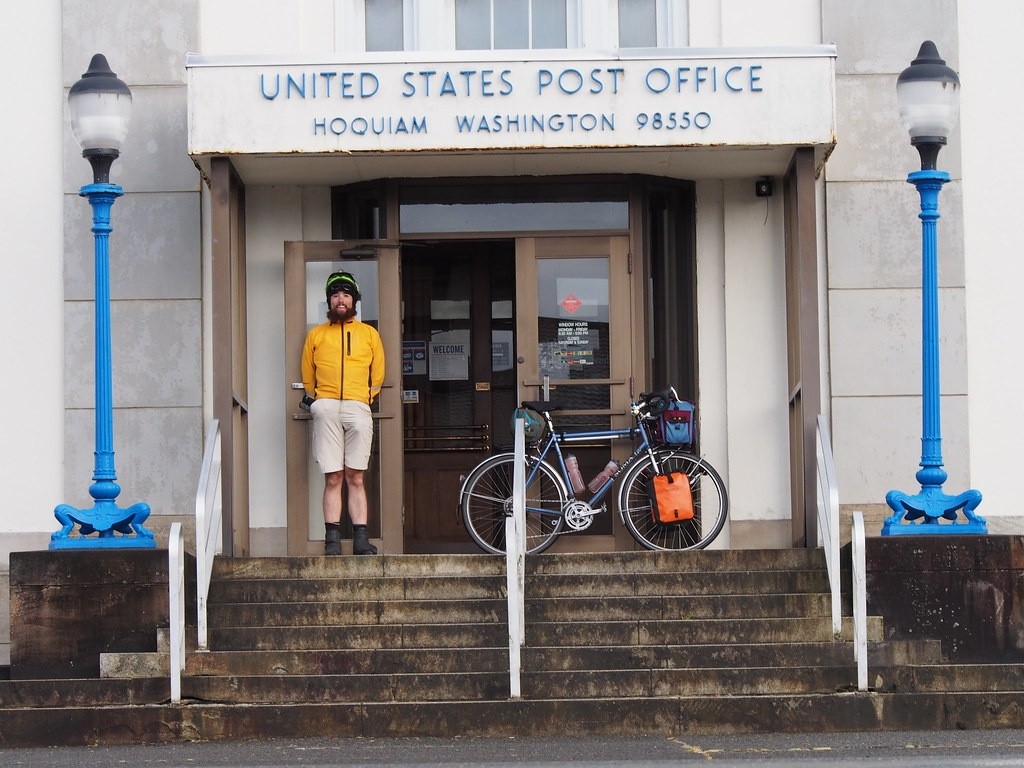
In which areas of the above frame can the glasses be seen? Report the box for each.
[331,284,352,293]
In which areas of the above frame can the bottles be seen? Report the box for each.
[564,452,586,494]
[588,459,621,494]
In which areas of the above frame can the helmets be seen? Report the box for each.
[325,271,361,301]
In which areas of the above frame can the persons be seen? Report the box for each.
[300,271,386,555]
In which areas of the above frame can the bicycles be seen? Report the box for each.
[457,385,728,556]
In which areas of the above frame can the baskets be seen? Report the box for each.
[651,398,699,445]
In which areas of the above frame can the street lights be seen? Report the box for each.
[52,53,157,549]
[881,40,987,536]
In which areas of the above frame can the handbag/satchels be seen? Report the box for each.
[646,468,695,524]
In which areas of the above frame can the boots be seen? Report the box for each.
[353,523,377,555]
[325,522,342,555]
